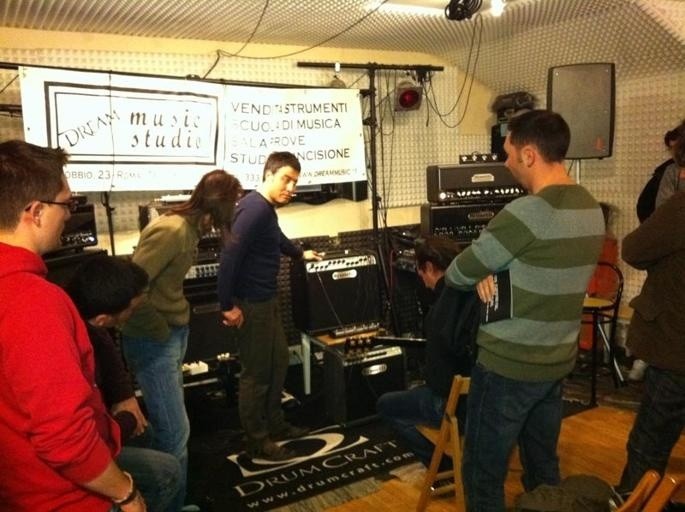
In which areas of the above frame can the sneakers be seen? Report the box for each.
[269,424,309,441]
[245,443,293,461]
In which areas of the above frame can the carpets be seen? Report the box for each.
[562,371,683,435]
[178,383,597,512]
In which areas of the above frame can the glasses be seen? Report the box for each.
[25,197,77,215]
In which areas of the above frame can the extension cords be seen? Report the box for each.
[328,320,380,339]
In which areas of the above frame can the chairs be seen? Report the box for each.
[414,375,471,512]
[567,262,623,406]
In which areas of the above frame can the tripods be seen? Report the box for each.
[576,159,629,387]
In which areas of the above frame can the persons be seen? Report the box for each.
[0,139,147,512]
[446,109,608,512]
[115,168,242,510]
[58,253,197,511]
[607,138,685,506]
[636,129,677,221]
[217,151,323,462]
[375,236,488,497]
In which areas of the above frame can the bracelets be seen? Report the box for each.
[111,470,137,505]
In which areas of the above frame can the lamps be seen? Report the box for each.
[395,78,423,112]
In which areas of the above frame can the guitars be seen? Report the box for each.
[344,337,426,354]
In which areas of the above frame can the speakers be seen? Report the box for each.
[289,250,382,337]
[323,340,405,430]
[546,63,615,160]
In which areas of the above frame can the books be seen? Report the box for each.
[477,268,512,326]
